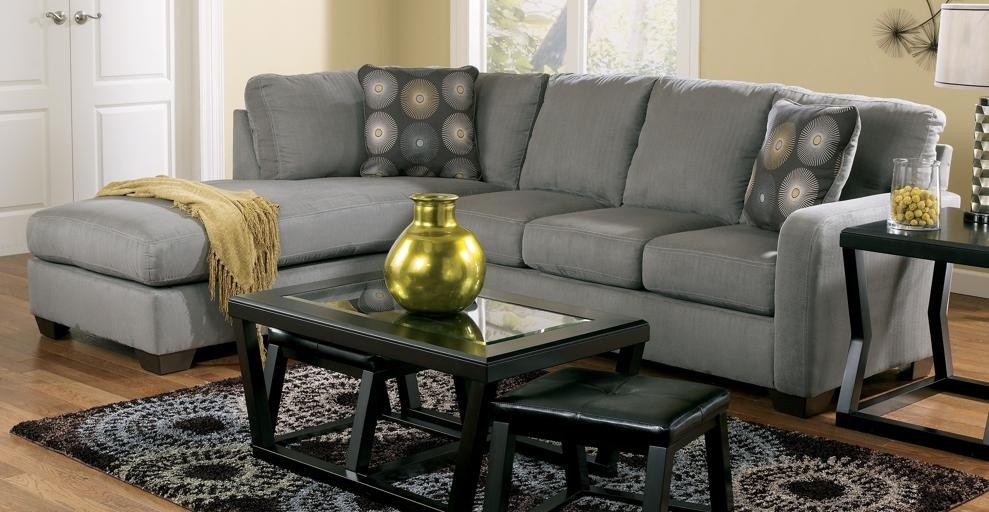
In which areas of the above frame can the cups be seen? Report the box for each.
[888,155,942,235]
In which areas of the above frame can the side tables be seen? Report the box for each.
[838,206,988,463]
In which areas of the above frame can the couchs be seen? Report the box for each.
[23,69,953,419]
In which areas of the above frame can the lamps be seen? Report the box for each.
[932,3,988,229]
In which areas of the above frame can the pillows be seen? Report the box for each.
[358,63,487,185]
[737,91,864,237]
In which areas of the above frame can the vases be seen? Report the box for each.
[381,192,485,318]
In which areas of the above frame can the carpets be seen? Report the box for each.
[9,365,989,511]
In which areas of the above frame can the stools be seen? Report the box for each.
[481,364,737,512]
